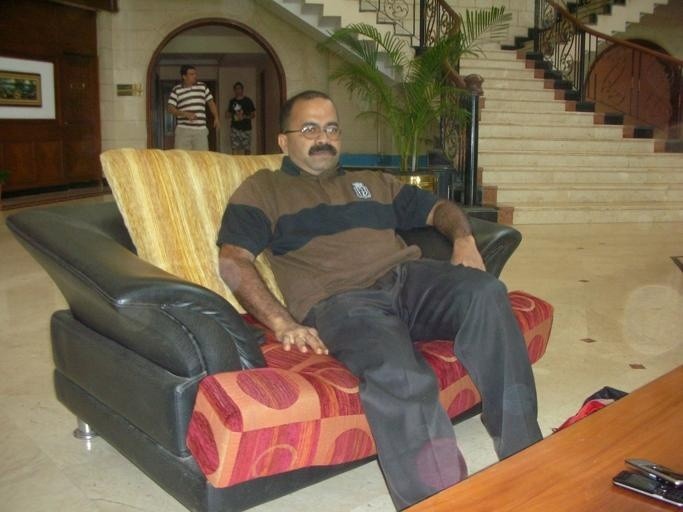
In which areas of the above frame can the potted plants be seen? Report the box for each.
[315,5,512,192]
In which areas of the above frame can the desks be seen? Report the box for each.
[396,367,682,510]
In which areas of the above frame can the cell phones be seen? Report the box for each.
[623,456,683,491]
[612,469,683,507]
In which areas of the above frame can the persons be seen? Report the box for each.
[167,65,220,151]
[225,82,257,155]
[216,90,543,512]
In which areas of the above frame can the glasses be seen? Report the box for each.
[286,122,341,142]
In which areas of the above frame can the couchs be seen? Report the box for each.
[5,201,555,512]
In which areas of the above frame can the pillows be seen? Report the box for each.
[96,147,291,314]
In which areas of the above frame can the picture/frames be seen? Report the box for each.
[0,52,60,124]
